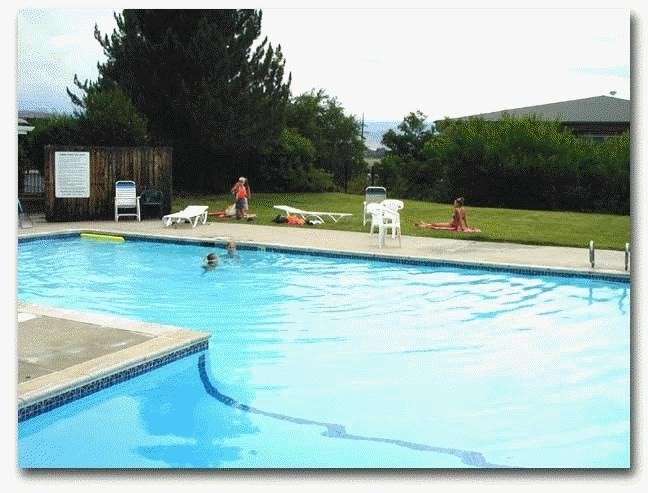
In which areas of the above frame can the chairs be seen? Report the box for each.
[363,184,405,248]
[273,204,354,224]
[114,179,164,221]
[162,204,209,226]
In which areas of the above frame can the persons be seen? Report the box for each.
[231,176,251,219]
[414,196,469,231]
[207,252,219,267]
[226,241,236,253]
